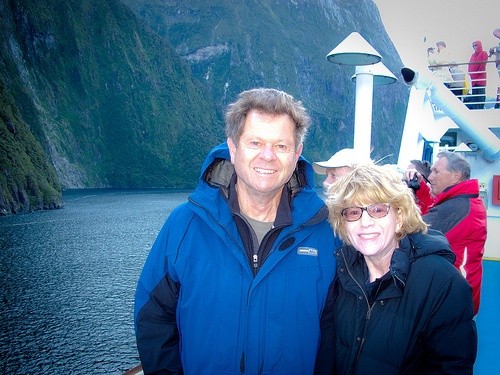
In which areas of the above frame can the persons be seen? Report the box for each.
[466,40,489,110]
[399,157,438,225]
[425,37,471,112]
[309,145,380,241]
[133,87,338,374]
[404,147,488,316]
[315,162,477,375]
[487,26,500,110]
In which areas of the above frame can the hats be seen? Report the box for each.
[312,148,376,175]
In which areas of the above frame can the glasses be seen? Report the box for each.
[340,202,391,222]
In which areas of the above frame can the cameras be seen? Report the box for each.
[488,48,494,57]
